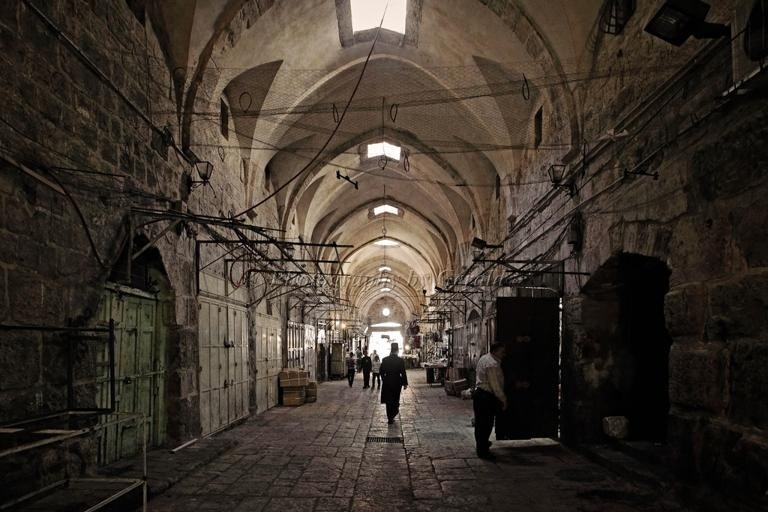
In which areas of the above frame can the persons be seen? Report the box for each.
[379,343,407,424]
[346,343,382,389]
[471,342,510,461]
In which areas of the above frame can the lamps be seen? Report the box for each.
[470,237,504,250]
[644,0,729,48]
[548,165,573,198]
[188,161,214,194]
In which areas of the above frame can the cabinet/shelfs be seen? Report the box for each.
[0,410,149,512]
